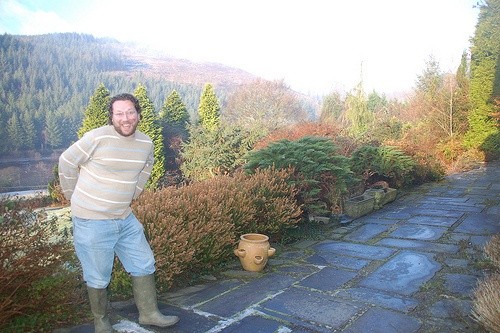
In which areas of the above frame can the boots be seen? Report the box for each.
[84,285,116,333]
[132,271,181,327]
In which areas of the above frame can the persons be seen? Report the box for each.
[57,93,180,333]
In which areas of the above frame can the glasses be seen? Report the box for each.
[111,108,138,117]
